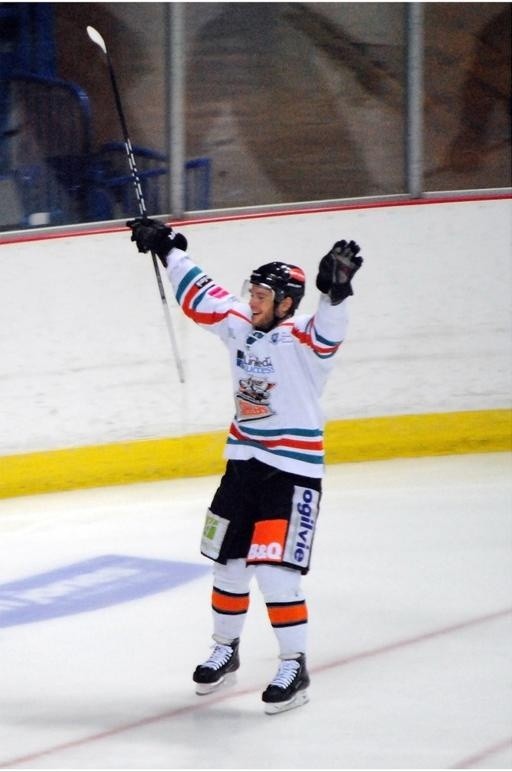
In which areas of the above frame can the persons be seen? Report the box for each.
[130,212,364,707]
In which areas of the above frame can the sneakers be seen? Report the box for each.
[193,636,241,684]
[261,653,310,701]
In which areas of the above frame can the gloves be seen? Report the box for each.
[315,239,363,305]
[125,217,187,268]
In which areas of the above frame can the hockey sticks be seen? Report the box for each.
[85,25,187,389]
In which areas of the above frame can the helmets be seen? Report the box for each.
[250,261,305,297]
